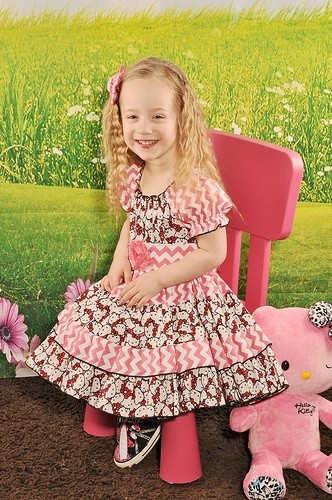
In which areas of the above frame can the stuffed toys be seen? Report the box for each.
[229,300,332,500]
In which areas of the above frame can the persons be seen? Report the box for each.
[24,56,290,470]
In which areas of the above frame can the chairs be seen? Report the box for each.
[82,126,305,485]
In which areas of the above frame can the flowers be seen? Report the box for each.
[106,64,126,105]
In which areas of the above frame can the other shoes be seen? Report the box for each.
[113,417,163,469]
